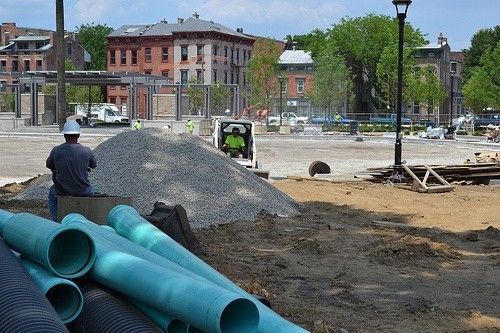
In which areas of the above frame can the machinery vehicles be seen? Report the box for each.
[213,117,269,182]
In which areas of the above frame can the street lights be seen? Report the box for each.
[386,1,414,183]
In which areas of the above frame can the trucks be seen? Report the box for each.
[69,101,129,123]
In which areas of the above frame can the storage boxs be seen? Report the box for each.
[56,195,133,225]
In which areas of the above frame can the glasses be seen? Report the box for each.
[233,132,239,134]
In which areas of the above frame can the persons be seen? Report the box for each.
[186,119,194,134]
[46,119,97,223]
[222,128,247,158]
[334,112,342,125]
[134,119,141,132]
[163,124,172,130]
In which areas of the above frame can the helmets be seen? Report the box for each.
[62,120,81,135]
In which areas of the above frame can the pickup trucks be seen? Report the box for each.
[266,111,309,125]
[369,113,413,126]
[310,114,354,124]
